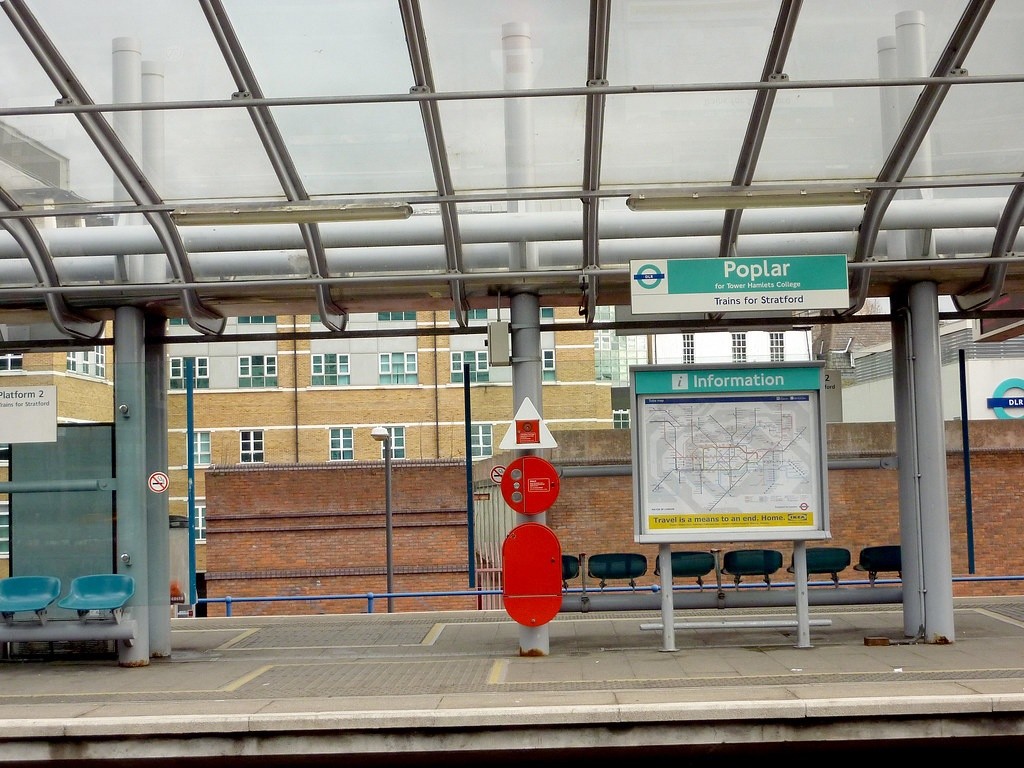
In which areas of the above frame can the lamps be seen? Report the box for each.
[169,202,413,225]
[626,186,872,211]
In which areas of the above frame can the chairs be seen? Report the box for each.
[588,553,648,594]
[787,547,851,587]
[854,545,902,586]
[721,550,783,590]
[0,575,62,627]
[654,551,716,590]
[58,573,136,624]
[562,553,586,595]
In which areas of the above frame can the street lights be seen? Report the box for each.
[371,427,394,613]
